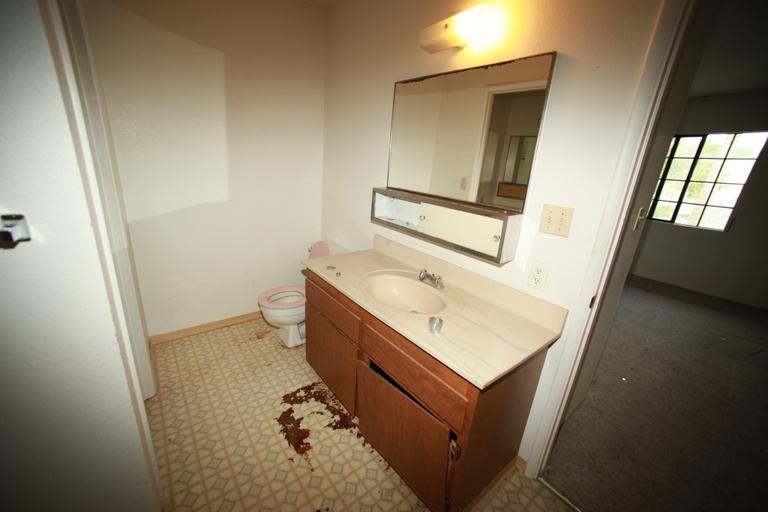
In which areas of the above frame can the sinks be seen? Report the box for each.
[362,272,447,316]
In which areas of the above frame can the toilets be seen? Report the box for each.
[258,238,350,348]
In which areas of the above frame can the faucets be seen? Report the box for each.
[418,268,442,288]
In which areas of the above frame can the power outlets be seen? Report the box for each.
[538,203,574,239]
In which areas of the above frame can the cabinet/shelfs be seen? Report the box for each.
[370,186,522,267]
[299,276,359,419]
[359,320,548,512]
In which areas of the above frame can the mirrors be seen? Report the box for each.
[385,50,558,216]
